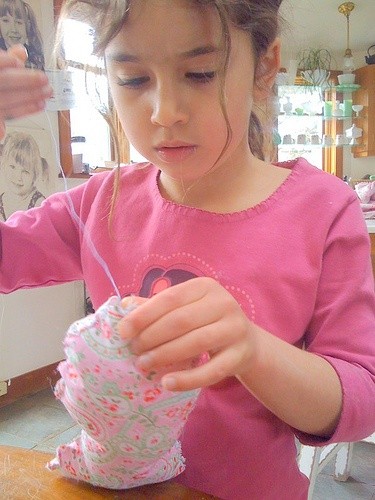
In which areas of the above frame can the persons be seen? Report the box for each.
[0,0,374,499]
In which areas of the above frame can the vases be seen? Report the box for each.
[271,60,364,147]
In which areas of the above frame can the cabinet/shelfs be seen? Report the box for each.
[352,64,375,158]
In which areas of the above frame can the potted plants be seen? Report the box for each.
[298,46,337,97]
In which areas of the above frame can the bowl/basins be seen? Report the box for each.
[337,74,356,84]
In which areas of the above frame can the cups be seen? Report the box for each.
[282,134,346,145]
[72,153,90,174]
